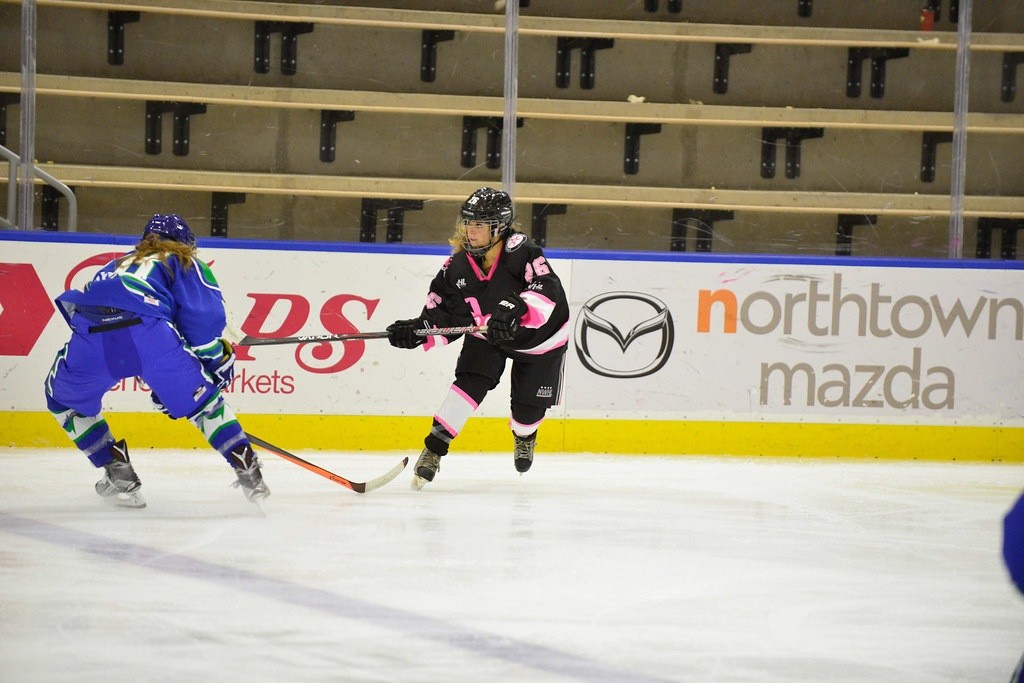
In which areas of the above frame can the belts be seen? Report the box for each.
[70,317,143,333]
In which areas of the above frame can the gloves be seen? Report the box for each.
[151,391,178,420]
[486,291,528,349]
[387,317,433,349]
[183,338,235,392]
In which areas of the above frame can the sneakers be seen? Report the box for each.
[229,444,274,515]
[95,438,147,508]
[408,417,454,490]
[512,429,538,477]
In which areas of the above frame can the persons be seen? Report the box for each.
[43,213,272,516]
[385,188,571,492]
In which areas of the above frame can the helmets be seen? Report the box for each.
[143,213,197,248]
[459,187,513,257]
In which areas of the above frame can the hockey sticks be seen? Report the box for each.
[242,429,410,494]
[223,307,490,348]
